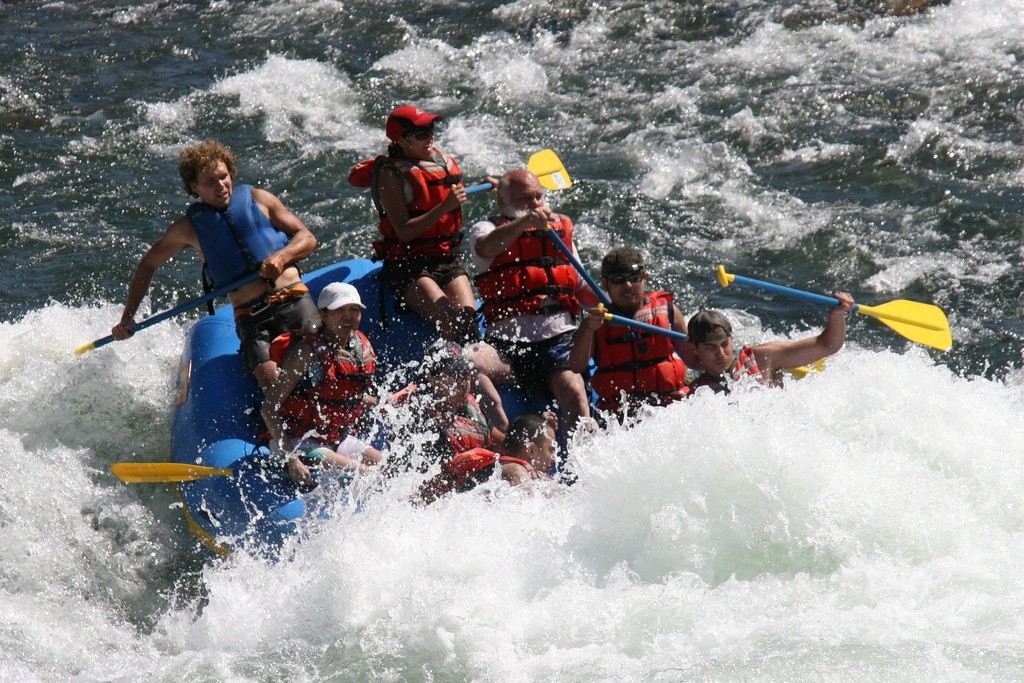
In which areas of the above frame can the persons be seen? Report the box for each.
[259,282,567,507]
[463,168,610,441]
[569,247,751,429]
[369,105,499,349]
[687,292,854,396]
[111,136,320,398]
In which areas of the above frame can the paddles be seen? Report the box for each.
[601,310,828,379]
[108,462,236,485]
[459,150,574,197]
[540,218,622,312]
[712,265,956,355]
[72,271,270,359]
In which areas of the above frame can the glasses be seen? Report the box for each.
[402,125,435,141]
[604,272,644,285]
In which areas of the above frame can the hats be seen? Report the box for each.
[386,106,443,142]
[688,310,732,343]
[317,282,367,311]
[601,247,645,276]
[422,341,477,377]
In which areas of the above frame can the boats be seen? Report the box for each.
[110,255,604,581]
